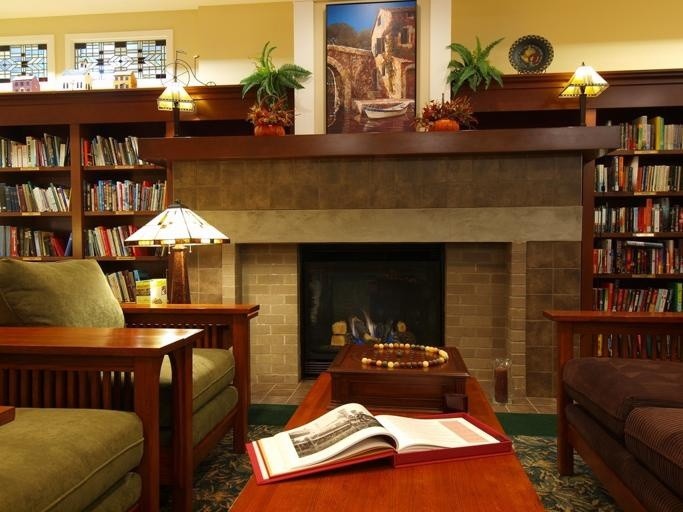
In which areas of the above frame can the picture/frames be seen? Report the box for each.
[326,1,416,132]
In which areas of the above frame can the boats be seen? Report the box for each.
[362,104,408,119]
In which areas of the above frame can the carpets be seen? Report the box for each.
[158,404,629,512]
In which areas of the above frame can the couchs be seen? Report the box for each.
[543,312,682,511]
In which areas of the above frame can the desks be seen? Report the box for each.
[227,369,547,512]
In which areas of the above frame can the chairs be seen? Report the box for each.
[0,338,184,512]
[1,258,260,512]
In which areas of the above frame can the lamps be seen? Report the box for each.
[124,200,229,303]
[157,78,195,138]
[559,59,610,125]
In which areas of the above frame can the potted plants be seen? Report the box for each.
[413,88,479,133]
[247,94,300,136]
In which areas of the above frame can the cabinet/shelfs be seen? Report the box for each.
[0,84,295,303]
[450,67,682,359]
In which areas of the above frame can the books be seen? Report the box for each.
[0,133,167,305]
[591,115,683,359]
[245,402,513,486]
